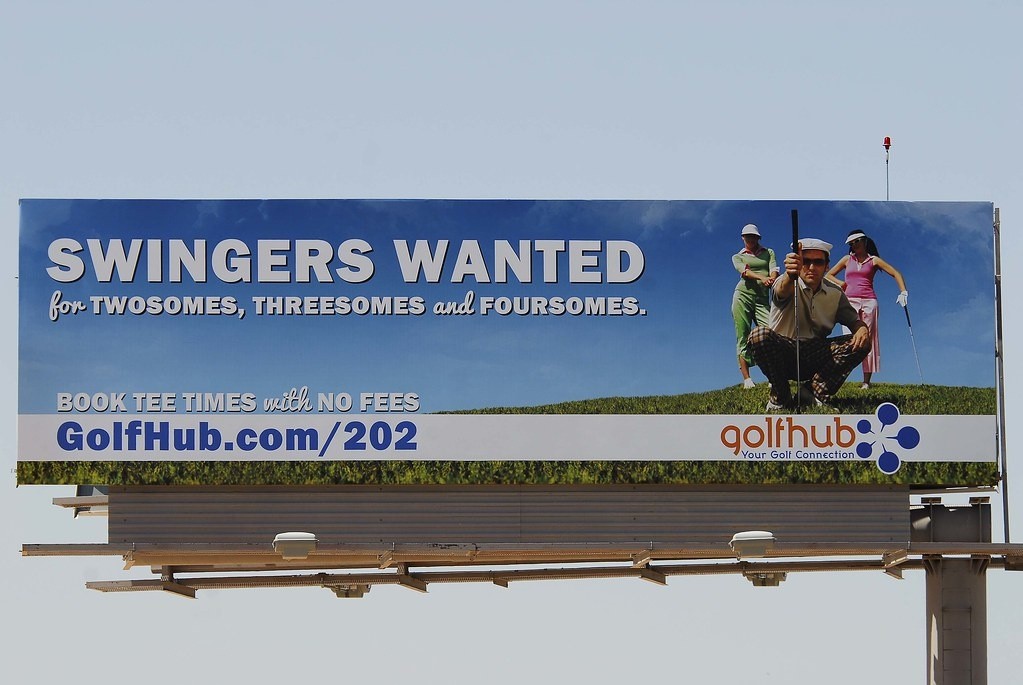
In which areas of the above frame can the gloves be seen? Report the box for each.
[896,291,909,307]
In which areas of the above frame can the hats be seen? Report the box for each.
[741,224,761,236]
[845,233,865,245]
[790,238,833,257]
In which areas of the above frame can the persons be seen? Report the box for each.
[824,229,909,391]
[731,224,781,389]
[745,237,873,413]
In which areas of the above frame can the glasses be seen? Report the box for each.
[848,238,865,247]
[803,258,830,267]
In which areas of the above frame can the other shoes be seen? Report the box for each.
[766,394,792,411]
[794,386,839,412]
[862,382,869,390]
[744,378,755,389]
[768,382,772,387]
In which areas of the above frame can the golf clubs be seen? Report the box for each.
[904,305,925,386]
[790,208,801,414]
